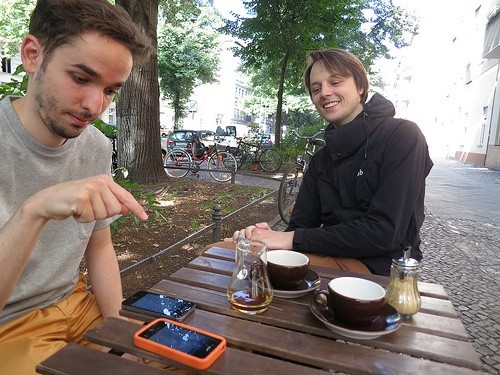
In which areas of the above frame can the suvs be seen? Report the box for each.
[166,129,231,157]
[160,124,169,138]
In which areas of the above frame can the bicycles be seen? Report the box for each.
[232,134,282,173]
[162,132,238,183]
[277,128,326,225]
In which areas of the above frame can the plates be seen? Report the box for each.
[307,290,402,340]
[248,262,322,299]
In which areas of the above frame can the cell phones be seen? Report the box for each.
[134,318,226,369]
[120,291,197,322]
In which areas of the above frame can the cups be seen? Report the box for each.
[313,277,386,329]
[258,250,310,291]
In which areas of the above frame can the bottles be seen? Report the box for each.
[383,246,421,319]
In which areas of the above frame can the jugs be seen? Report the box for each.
[226,237,273,315]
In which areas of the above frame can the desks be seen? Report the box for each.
[35,245,483,375]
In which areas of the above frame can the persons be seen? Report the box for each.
[0,0,192,375]
[202,47,434,277]
